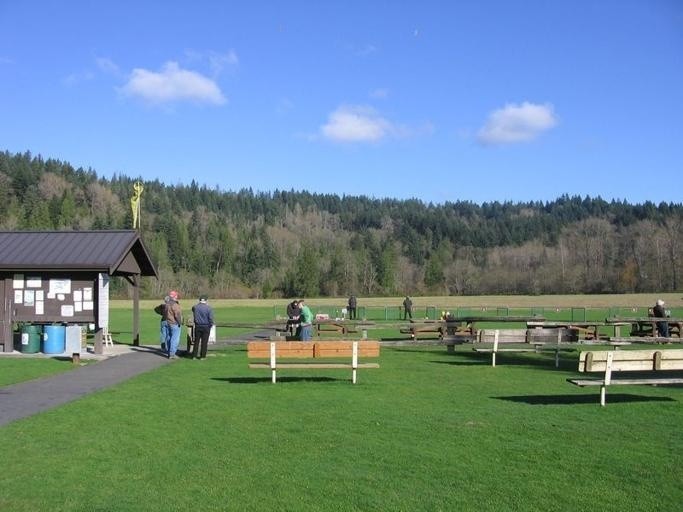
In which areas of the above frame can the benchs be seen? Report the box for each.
[247,341,380,384]
[254,313,375,341]
[87,333,113,347]
[567,350,683,406]
[400,313,682,367]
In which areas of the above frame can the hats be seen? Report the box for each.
[200,294,207,300]
[656,300,665,306]
[169,291,177,297]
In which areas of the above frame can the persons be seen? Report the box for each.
[192,294,213,360]
[155,291,182,359]
[403,296,412,320]
[287,300,313,341]
[653,299,672,345]
[349,295,356,320]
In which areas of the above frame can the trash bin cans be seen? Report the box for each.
[21,325,87,354]
